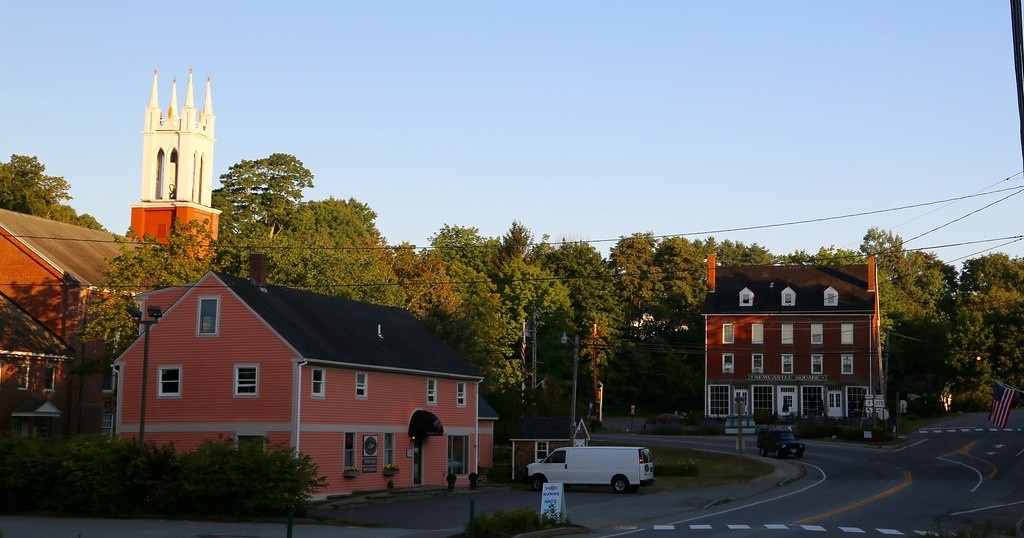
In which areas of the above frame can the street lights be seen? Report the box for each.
[560,335,579,447]
[735,397,745,460]
[125,305,163,449]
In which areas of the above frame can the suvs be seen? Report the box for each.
[757,428,807,460]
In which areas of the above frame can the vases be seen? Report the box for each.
[344,471,360,476]
[383,468,399,474]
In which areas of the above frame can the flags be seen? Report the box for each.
[520,329,527,379]
[987,381,1017,429]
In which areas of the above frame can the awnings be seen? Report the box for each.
[408,410,443,437]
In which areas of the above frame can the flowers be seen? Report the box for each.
[347,466,360,471]
[386,463,400,470]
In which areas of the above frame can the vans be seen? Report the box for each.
[525,444,655,493]
